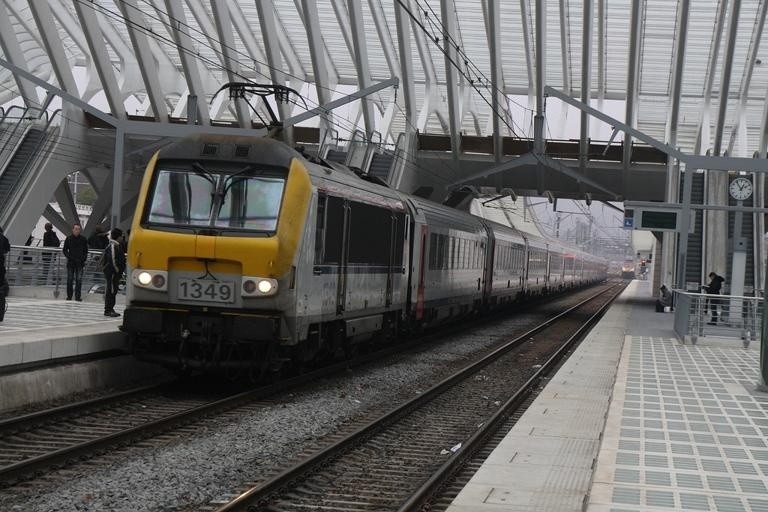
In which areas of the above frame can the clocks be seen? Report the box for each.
[729,176,753,201]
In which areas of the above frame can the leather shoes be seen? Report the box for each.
[75,297,82,301]
[66,296,72,300]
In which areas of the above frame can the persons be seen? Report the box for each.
[0,225,11,299]
[102,228,126,318]
[701,272,725,325]
[655,284,673,312]
[88,224,109,284]
[103,228,132,254]
[62,222,89,302]
[41,222,61,285]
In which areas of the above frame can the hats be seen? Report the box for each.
[111,228,122,240]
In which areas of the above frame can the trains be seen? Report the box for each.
[620,259,635,278]
[110,130,610,390]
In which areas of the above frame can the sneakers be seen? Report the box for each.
[104,309,117,317]
